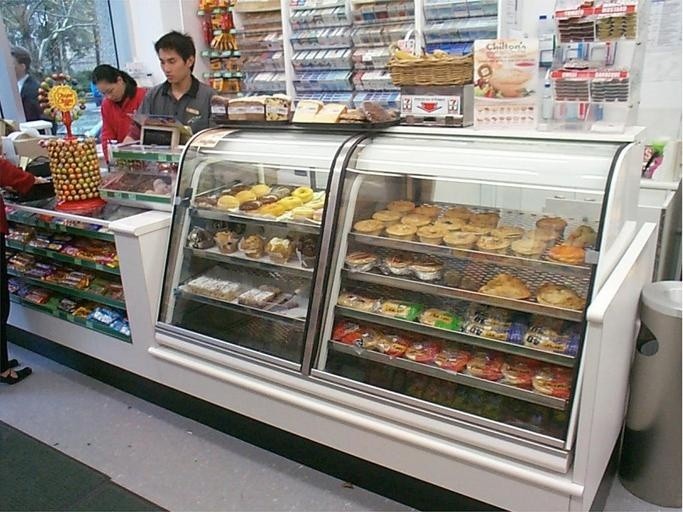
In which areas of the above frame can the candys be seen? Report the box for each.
[33,72,103,202]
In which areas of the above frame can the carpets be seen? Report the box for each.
[0,420,168,512]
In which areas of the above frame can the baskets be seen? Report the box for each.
[385,51,473,86]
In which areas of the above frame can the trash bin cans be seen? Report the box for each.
[618,280,683,507]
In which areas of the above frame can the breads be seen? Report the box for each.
[433,48,448,59]
[396,50,417,60]
[338,198,597,310]
[210,95,391,124]
[182,182,325,310]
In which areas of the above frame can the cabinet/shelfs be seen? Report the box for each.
[0,168,171,411]
[196,1,524,114]
[146,124,658,511]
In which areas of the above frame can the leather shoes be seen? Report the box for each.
[6,359,19,368]
[0,365,33,385]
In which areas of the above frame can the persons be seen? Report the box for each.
[93,65,150,171]
[123,31,217,143]
[11,45,58,134]
[91,81,105,143]
[1,158,47,385]
